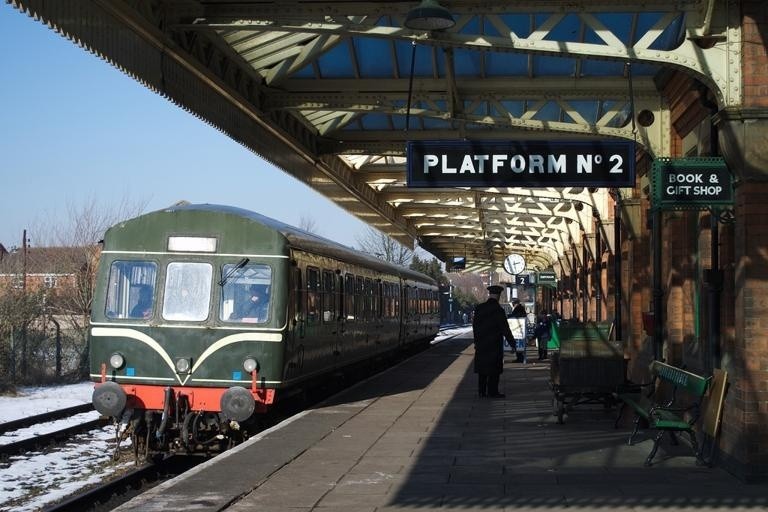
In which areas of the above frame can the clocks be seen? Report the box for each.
[503,254,526,275]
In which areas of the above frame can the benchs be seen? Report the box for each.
[543,315,626,426]
[611,356,715,467]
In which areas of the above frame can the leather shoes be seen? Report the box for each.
[480,391,507,399]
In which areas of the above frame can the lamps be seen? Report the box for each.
[404,1,457,31]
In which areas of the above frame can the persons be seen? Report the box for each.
[534,311,552,360]
[456,310,475,325]
[508,298,527,363]
[236,284,268,319]
[472,286,516,399]
[130,287,153,317]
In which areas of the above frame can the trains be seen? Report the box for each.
[86,201,441,466]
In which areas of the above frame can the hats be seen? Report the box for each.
[487,285,505,295]
